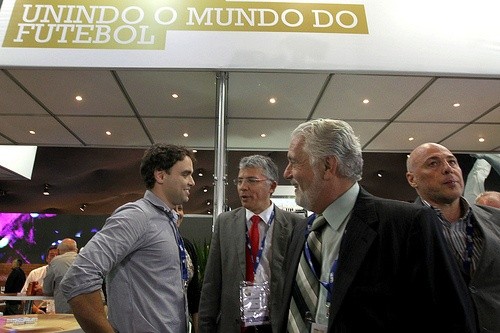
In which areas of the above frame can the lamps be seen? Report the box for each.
[42,184,50,196]
[80,203,88,212]
[198,168,212,214]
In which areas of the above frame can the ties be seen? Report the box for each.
[244,215,263,282]
[288,216,330,333]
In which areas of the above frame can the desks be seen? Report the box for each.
[0,314,109,333]
[0,295,54,315]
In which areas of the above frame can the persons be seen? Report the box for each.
[41,237,79,314]
[405,139,500,333]
[198,151,307,332]
[58,142,195,333]
[272,118,480,332]
[3,257,28,316]
[472,190,500,211]
[19,246,62,313]
[165,202,206,333]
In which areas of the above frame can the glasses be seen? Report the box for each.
[233,177,269,186]
